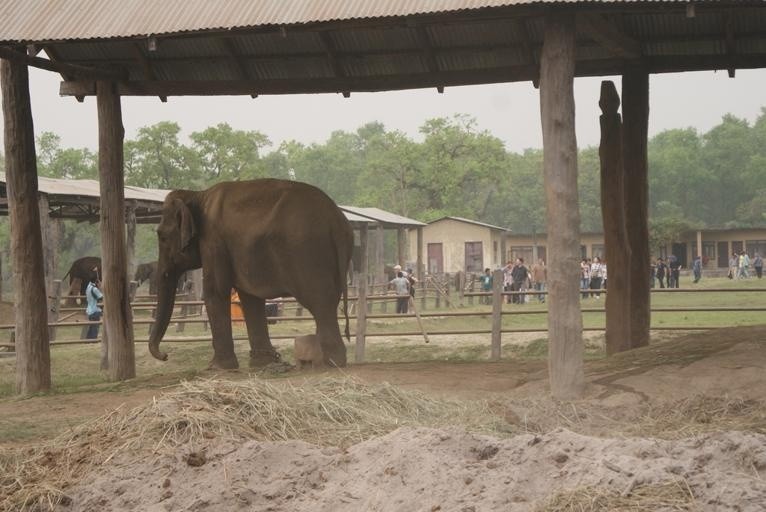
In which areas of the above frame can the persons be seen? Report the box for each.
[404,268,418,314]
[228,286,246,330]
[385,272,410,314]
[478,251,764,305]
[201,294,209,331]
[85,271,104,339]
[265,296,283,325]
[393,265,408,278]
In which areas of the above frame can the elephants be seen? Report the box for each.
[148,177,355,375]
[62,256,101,307]
[134,260,186,295]
